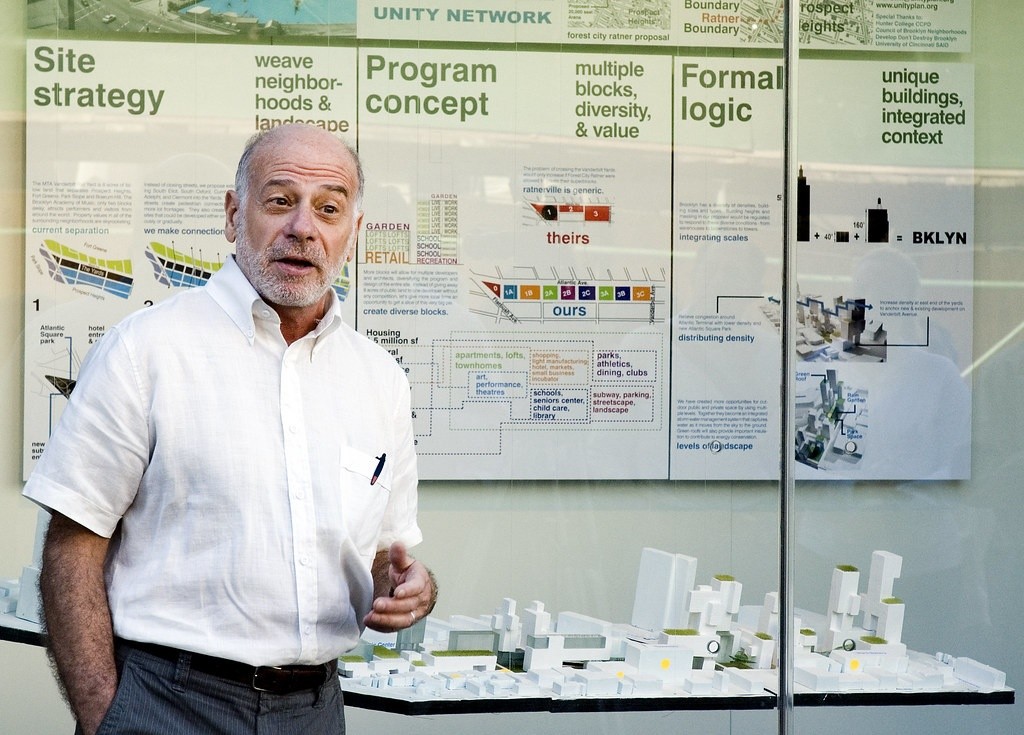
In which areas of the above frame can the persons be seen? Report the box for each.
[21,123,438,735]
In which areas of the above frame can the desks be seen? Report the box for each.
[0,610,1017,735]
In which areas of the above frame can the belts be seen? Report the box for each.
[115,636,339,693]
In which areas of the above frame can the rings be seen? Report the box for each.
[411,611,416,625]
